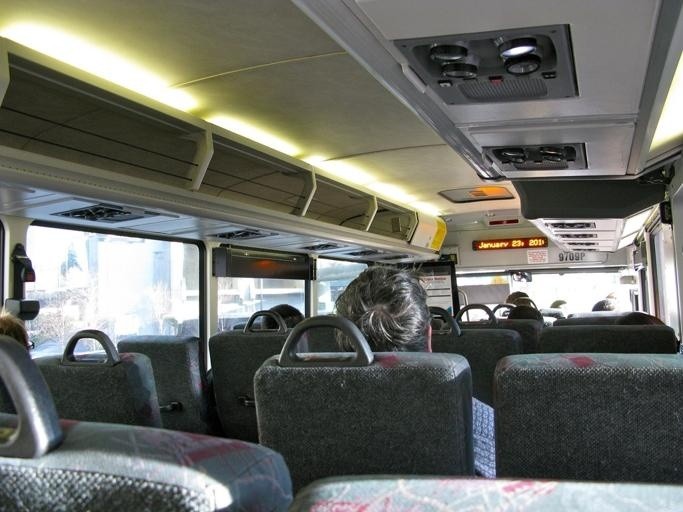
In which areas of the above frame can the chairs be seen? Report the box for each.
[0,297,683,512]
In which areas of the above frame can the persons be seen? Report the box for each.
[592,299,614,310]
[0,312,34,414]
[508,305,547,328]
[261,304,304,330]
[617,312,665,326]
[334,265,495,478]
[506,292,532,308]
